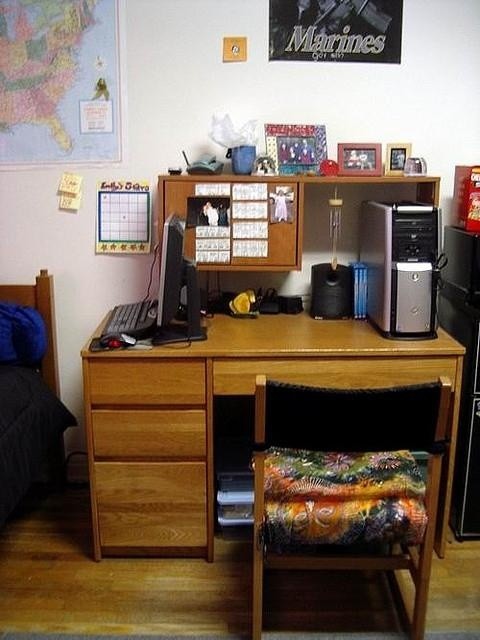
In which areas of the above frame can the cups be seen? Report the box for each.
[232,144,256,177]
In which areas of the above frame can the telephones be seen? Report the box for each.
[182,150,224,175]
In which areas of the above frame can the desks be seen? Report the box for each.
[80,174,466,563]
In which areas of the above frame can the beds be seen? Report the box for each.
[0,268,79,530]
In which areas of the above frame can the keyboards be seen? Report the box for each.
[102,299,159,339]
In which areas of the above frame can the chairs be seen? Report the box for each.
[250,375,452,640]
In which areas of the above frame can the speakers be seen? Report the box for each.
[311,262,355,322]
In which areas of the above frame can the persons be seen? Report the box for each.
[300,140,315,159]
[300,148,311,164]
[272,191,291,221]
[285,147,297,165]
[216,204,229,226]
[348,150,360,168]
[294,142,301,160]
[347,151,360,166]
[197,203,210,225]
[359,148,373,170]
[208,199,220,227]
[280,143,290,163]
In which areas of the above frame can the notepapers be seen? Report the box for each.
[58,173,82,194]
[60,192,81,210]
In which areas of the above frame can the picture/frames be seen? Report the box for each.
[264,123,328,176]
[384,141,412,178]
[337,143,381,177]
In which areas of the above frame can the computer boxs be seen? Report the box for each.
[360,200,443,341]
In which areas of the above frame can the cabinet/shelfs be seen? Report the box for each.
[437,224,480,544]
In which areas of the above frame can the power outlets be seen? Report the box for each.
[223,36,246,63]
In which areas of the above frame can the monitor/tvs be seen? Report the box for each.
[150,211,208,345]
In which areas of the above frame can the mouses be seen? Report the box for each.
[100,332,136,347]
